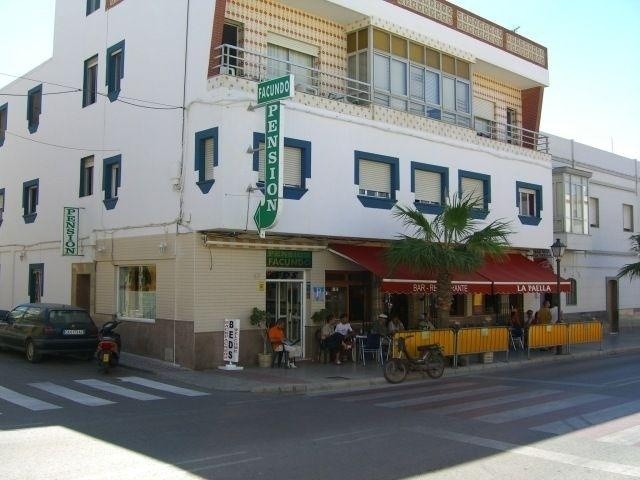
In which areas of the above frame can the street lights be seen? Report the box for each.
[549,237,568,352]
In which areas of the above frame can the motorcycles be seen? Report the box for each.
[382,334,445,383]
[97,320,123,371]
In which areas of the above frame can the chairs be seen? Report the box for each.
[266,329,296,369]
[507,326,524,351]
[318,334,392,368]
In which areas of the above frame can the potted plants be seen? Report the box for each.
[250,306,275,367]
[310,308,334,363]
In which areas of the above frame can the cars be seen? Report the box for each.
[0,303,97,364]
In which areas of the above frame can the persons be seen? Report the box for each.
[320,312,438,365]
[268,319,297,368]
[509,299,553,351]
